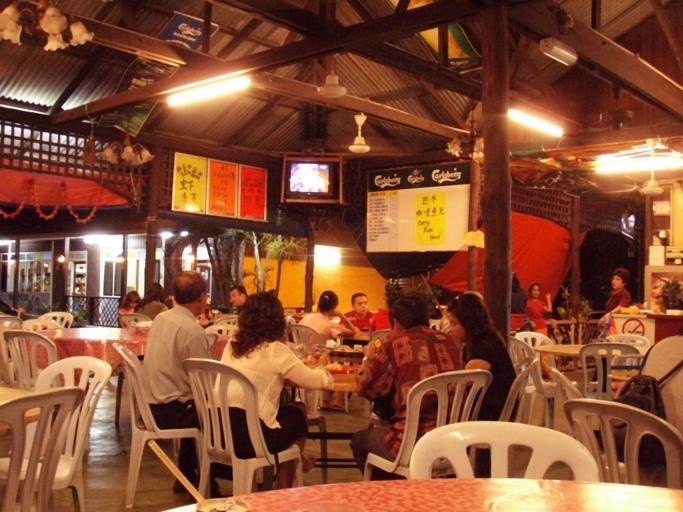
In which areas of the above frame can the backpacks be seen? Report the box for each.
[598,344,683,467]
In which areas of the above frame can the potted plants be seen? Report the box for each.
[653,276,683,315]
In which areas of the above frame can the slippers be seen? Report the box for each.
[321,398,351,411]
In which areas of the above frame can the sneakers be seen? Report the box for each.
[173,473,219,494]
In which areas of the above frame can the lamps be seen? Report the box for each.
[445,101,484,164]
[539,36,578,67]
[99,106,155,167]
[0,0,95,52]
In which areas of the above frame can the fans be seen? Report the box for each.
[314,54,346,99]
[334,111,394,154]
[642,173,664,197]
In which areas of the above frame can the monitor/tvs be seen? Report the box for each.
[279,156,346,206]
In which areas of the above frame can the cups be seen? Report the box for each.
[353,345,362,352]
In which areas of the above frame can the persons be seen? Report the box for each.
[592,268,631,393]
[512,271,527,331]
[638,325,682,489]
[349,291,467,481]
[119,282,248,331]
[525,282,553,379]
[139,272,223,494]
[208,292,333,488]
[445,291,519,471]
[289,283,405,351]
